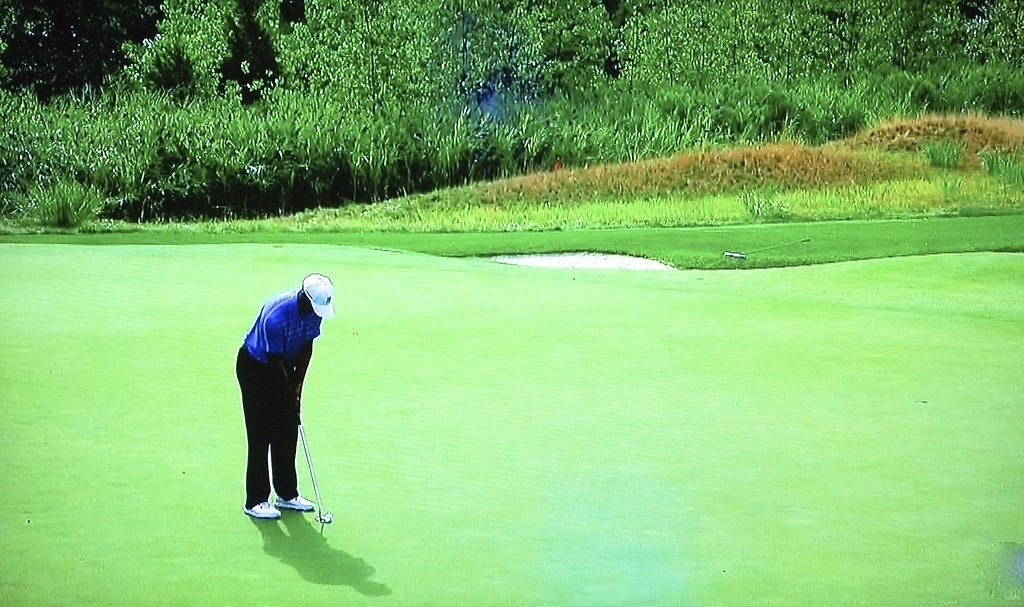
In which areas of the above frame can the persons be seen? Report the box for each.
[236,273,335,518]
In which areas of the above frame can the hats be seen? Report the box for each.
[302,272,334,317]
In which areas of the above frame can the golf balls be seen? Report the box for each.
[325,513,333,523]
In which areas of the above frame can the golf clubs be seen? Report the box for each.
[298,416,331,523]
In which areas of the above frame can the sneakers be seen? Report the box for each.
[274,494,314,511]
[242,502,281,518]
